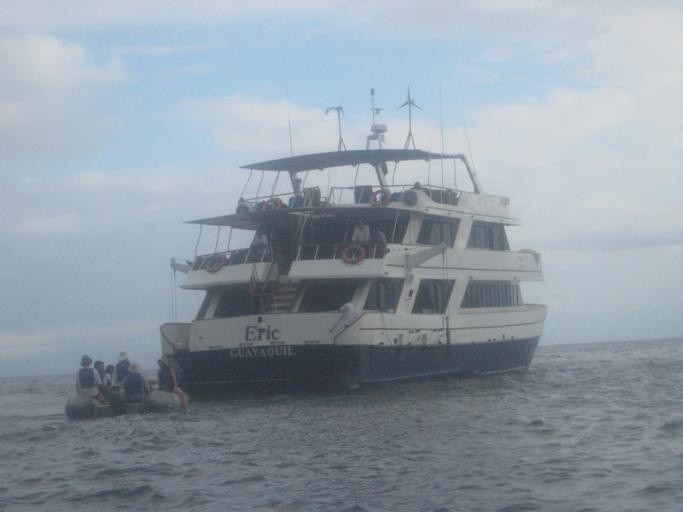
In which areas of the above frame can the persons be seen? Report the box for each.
[158,355,189,414]
[236,198,250,214]
[352,218,369,243]
[373,229,387,244]
[75,349,150,403]
[339,298,353,328]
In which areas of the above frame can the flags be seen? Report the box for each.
[248,267,258,297]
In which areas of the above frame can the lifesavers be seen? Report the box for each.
[341,244,365,264]
[369,189,392,207]
[204,255,227,272]
[263,198,283,211]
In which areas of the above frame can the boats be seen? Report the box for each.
[63,382,187,421]
[158,86,548,395]
